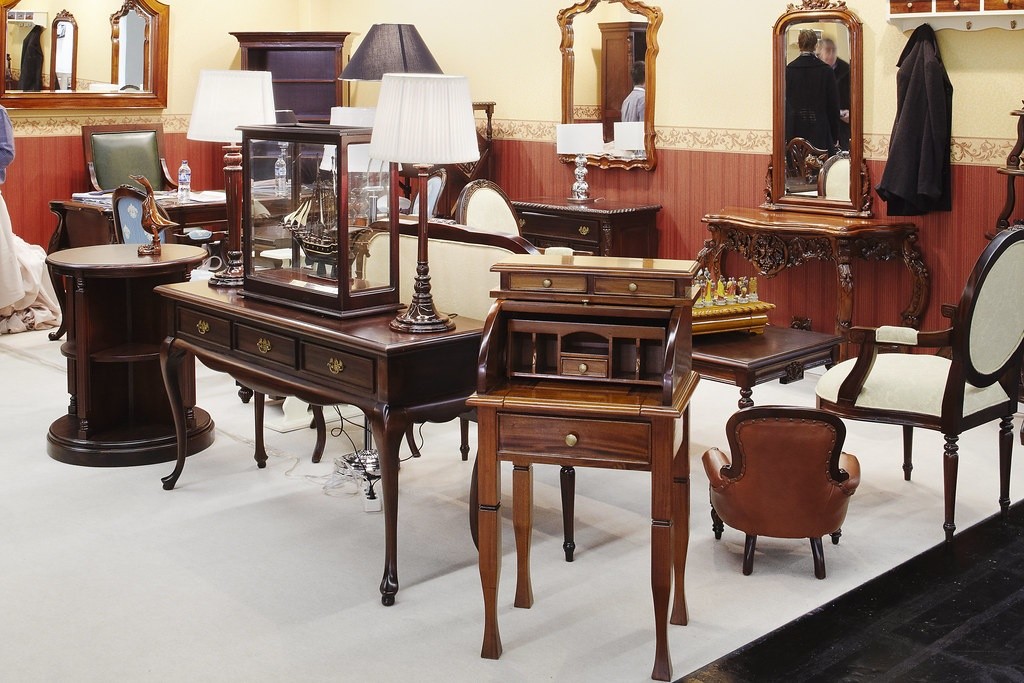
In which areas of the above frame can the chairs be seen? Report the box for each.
[810,226,1023,542]
[408,170,524,458]
[701,404,860,577]
[82,123,198,196]
[787,137,851,204]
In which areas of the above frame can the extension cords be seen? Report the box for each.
[357,479,381,512]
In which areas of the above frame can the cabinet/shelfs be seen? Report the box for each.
[886,0,1024,35]
[467,254,700,679]
[42,243,216,469]
[4,10,48,29]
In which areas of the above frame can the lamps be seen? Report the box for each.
[553,123,604,203]
[613,121,648,152]
[189,24,480,336]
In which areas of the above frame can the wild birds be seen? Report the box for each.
[129,174,180,255]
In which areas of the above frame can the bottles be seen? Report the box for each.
[178,160,191,202]
[274,156,287,195]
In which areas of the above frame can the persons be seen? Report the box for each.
[620,61,646,159]
[784,30,852,161]
[693,268,758,303]
[0,104,26,314]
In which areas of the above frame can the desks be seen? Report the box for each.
[700,206,929,370]
[507,195,662,259]
[688,327,842,403]
[151,263,487,606]
[47,189,287,341]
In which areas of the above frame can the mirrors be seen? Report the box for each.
[771,0,868,212]
[0,0,170,107]
[110,1,150,91]
[49,8,77,92]
[556,1,663,171]
